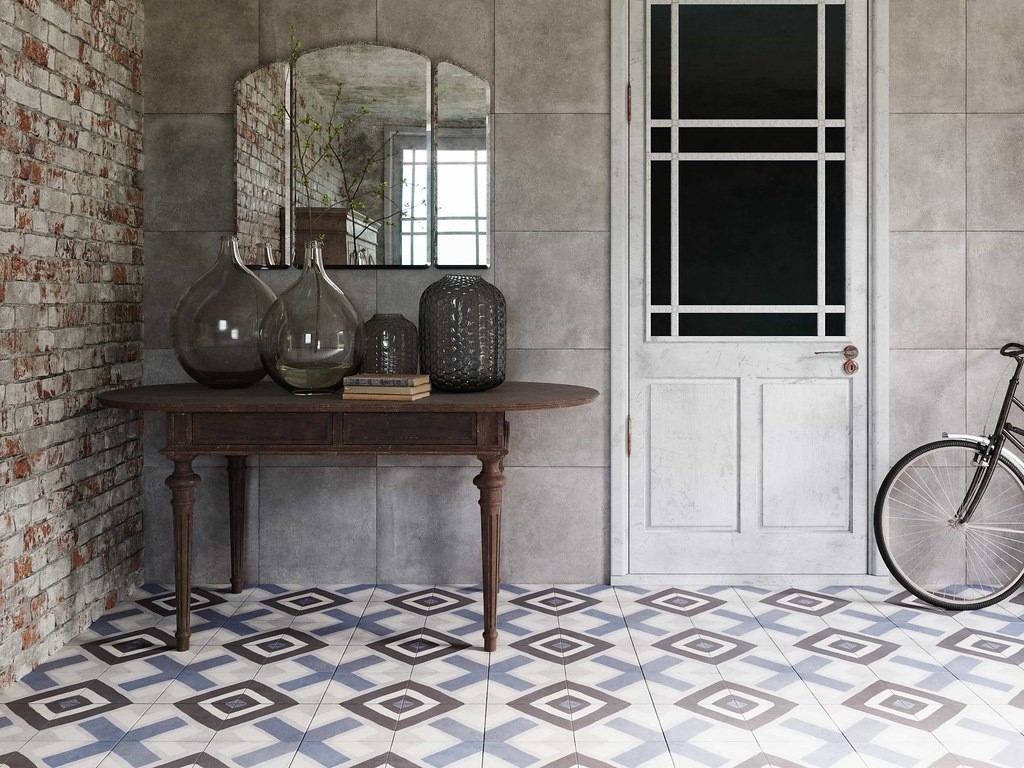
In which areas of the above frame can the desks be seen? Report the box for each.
[97,380,600,654]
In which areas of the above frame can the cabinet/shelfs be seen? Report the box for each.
[278,207,382,265]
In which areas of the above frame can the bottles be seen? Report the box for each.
[171,236,288,389]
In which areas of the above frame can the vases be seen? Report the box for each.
[169,234,289,389]
[258,240,366,395]
[417,274,507,392]
[353,313,419,375]
[350,252,363,265]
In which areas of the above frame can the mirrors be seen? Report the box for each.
[231,39,492,270]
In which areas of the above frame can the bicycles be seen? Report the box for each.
[873,343,1024,611]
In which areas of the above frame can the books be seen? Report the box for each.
[340,373,432,400]
